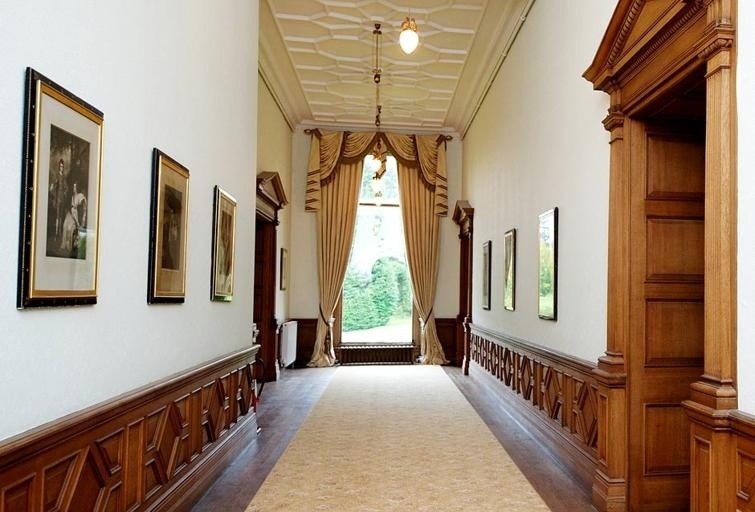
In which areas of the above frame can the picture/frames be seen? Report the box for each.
[17,65,105,314]
[210,186,238,303]
[147,148,190,310]
[481,208,557,322]
[279,247,289,290]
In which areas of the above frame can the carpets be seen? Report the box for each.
[239,364,551,511]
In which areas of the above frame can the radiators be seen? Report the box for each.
[281,320,300,368]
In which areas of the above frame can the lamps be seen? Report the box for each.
[398,2,419,56]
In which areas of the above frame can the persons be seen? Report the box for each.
[49,158,87,253]
